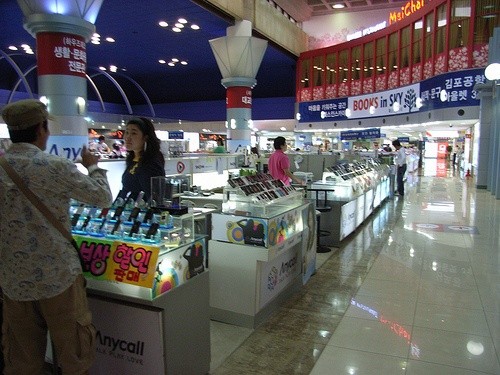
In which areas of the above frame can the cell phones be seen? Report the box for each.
[327,161,372,180]
[71,183,173,239]
[227,172,296,201]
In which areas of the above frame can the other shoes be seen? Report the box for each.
[394,193,400,195]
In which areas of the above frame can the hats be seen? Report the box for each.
[2,99,56,130]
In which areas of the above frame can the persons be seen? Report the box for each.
[293,148,302,152]
[250,147,259,158]
[121,118,166,203]
[1,99,113,375]
[392,139,407,196]
[93,135,128,159]
[317,141,392,154]
[452,144,459,165]
[213,138,226,153]
[267,136,307,188]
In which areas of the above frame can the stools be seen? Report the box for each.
[302,189,335,254]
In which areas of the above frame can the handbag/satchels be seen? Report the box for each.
[78,250,86,272]
